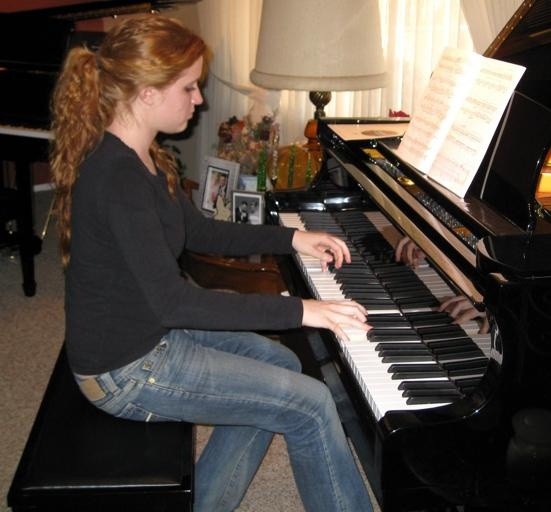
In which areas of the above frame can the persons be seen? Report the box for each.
[394,235,492,334]
[214,173,227,209]
[235,200,260,224]
[46,12,373,512]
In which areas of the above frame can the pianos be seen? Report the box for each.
[265,0,550,511]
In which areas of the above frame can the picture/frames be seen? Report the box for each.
[231,190,266,225]
[196,155,241,223]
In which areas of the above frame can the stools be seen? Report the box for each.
[6,337,195,512]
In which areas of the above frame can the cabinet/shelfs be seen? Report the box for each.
[182,248,287,296]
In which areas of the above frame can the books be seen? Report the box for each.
[395,48,527,199]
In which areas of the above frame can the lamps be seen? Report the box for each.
[249,0,392,149]
